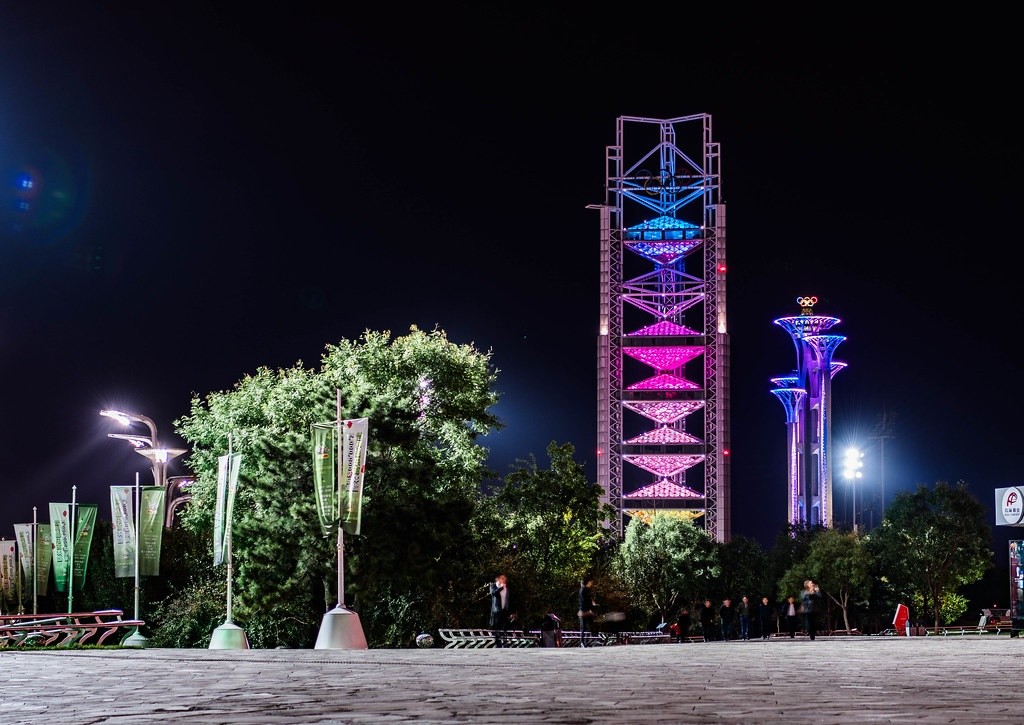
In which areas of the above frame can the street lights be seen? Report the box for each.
[99,409,189,486]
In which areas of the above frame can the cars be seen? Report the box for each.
[884,629,897,635]
[830,630,862,636]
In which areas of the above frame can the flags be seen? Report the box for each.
[1,418,370,607]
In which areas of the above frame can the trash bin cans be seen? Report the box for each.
[919,627,924,636]
[910,627,917,636]
[542,614,562,648]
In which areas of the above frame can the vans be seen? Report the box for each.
[774,632,807,637]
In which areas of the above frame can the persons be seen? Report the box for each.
[782,596,800,638]
[670,606,691,643]
[490,575,514,649]
[737,595,755,641]
[577,577,599,647]
[758,597,772,640]
[699,599,714,642]
[802,581,824,642]
[719,598,736,642]
